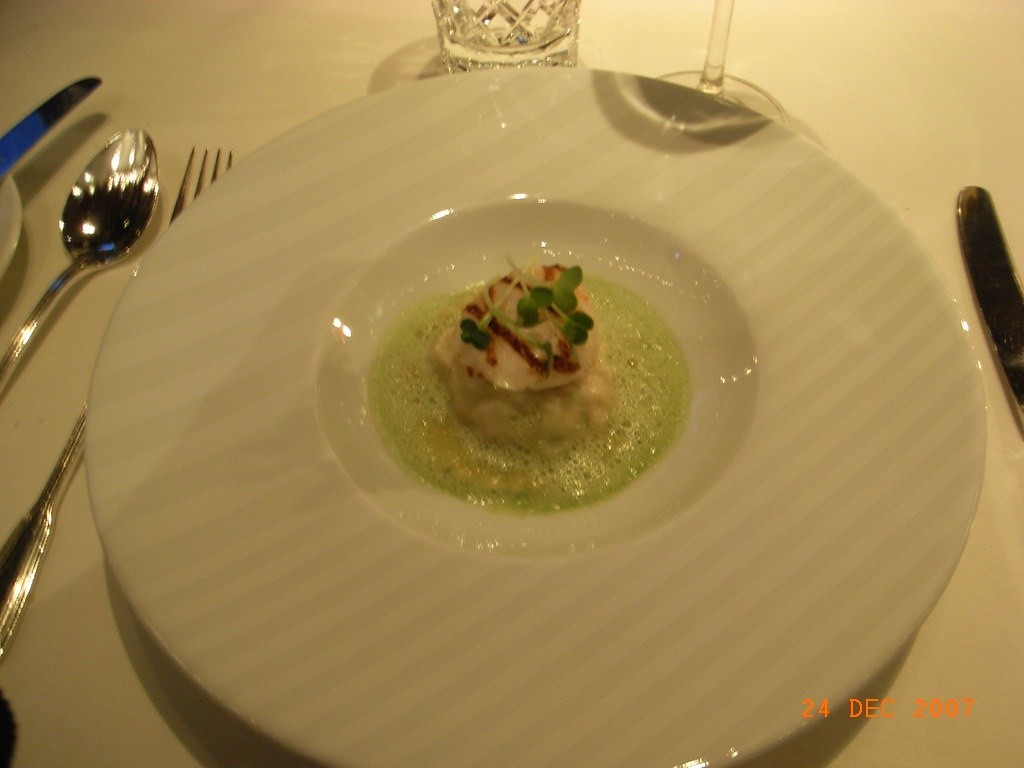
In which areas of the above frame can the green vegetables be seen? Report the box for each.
[458,252,594,376]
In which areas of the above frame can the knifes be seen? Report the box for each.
[956,184,1024,429]
[0,77,102,182]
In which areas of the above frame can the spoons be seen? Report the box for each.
[0,129,159,394]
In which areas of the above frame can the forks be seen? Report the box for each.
[0,146,236,664]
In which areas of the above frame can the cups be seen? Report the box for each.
[432,0,581,73]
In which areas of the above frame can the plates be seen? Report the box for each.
[0,174,22,273]
[87,65,987,768]
